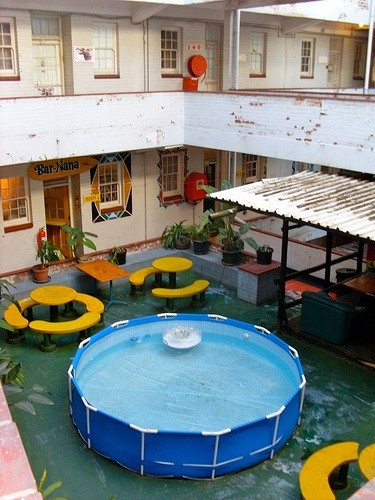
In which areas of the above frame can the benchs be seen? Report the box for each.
[4,298,105,353]
[129,267,163,296]
[300,442,358,500]
[151,279,210,311]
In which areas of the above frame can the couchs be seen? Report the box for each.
[299,291,368,345]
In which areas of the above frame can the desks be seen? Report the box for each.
[29,286,77,322]
[343,272,375,306]
[152,256,193,289]
[74,259,131,312]
[358,444,375,481]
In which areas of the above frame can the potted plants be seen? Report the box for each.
[162,219,191,250]
[256,244,273,265]
[190,222,211,255]
[109,248,127,266]
[197,179,259,264]
[60,224,98,264]
[31,240,59,281]
